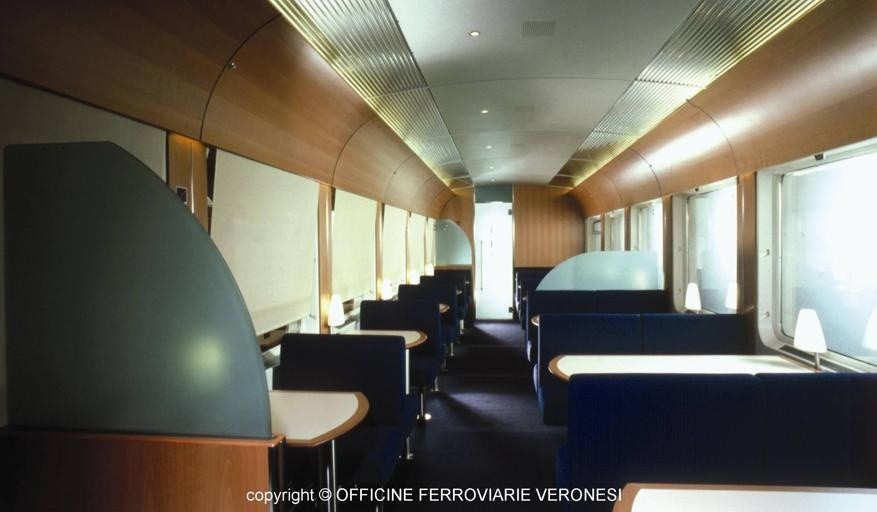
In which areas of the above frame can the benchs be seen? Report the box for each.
[509,264,875,512]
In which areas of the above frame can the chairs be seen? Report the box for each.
[266,267,474,512]
